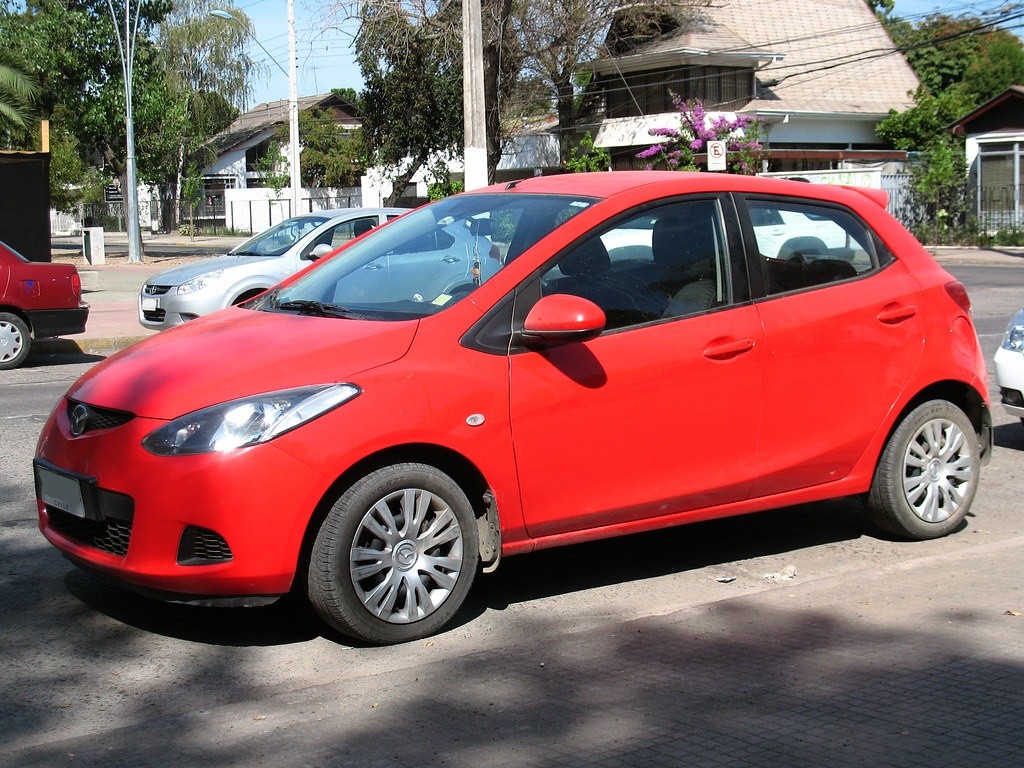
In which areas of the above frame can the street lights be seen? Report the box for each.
[209,9,302,217]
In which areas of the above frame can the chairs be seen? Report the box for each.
[313,227,335,247]
[542,236,623,330]
[354,219,376,238]
[629,216,718,320]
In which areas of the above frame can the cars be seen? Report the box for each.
[540,198,873,287]
[0,238,90,371]
[132,204,506,336]
[31,167,997,649]
